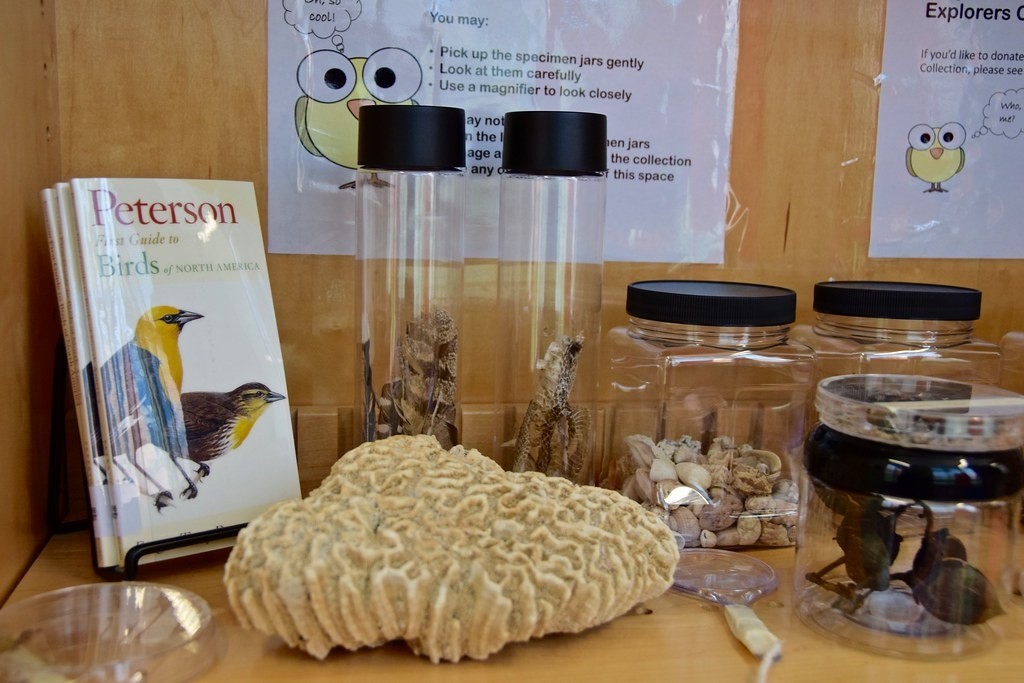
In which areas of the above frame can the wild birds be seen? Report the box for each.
[84,306,206,515]
[149,381,286,500]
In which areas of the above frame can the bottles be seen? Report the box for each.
[349,99,468,454]
[493,107,608,486]
[599,274,817,549]
[790,375,1024,664]
[789,277,1002,498]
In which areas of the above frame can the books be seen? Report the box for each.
[40,178,303,566]
